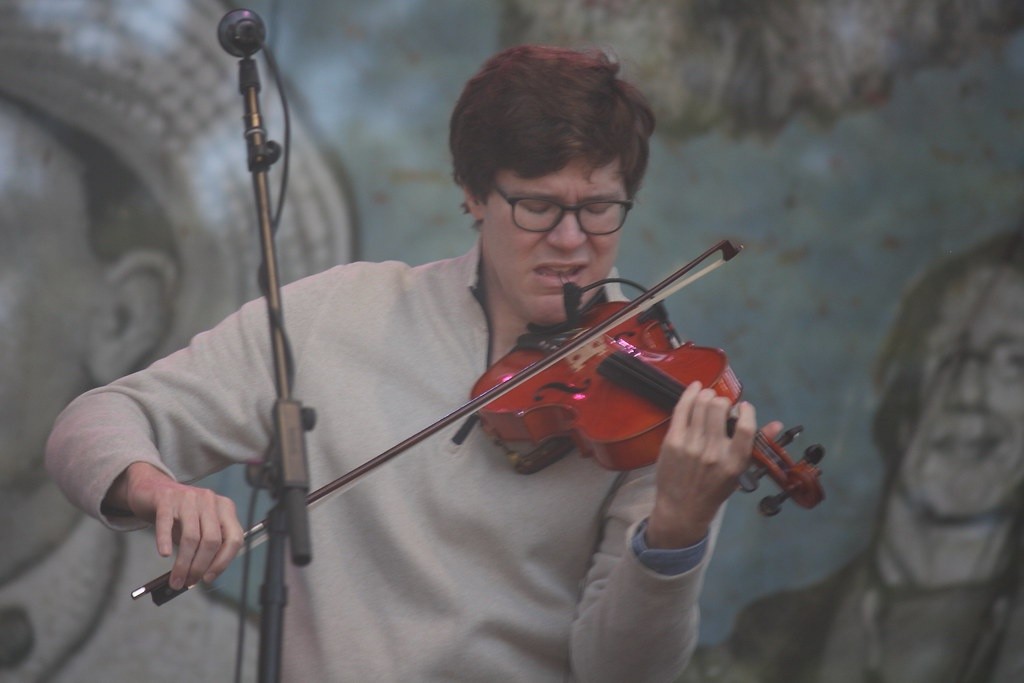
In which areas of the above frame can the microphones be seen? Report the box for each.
[220,8,266,55]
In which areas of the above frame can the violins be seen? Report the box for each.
[470,300,825,516]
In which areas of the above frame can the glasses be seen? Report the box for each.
[489,178,634,238]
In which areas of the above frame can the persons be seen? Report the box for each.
[45,47,785,683]
